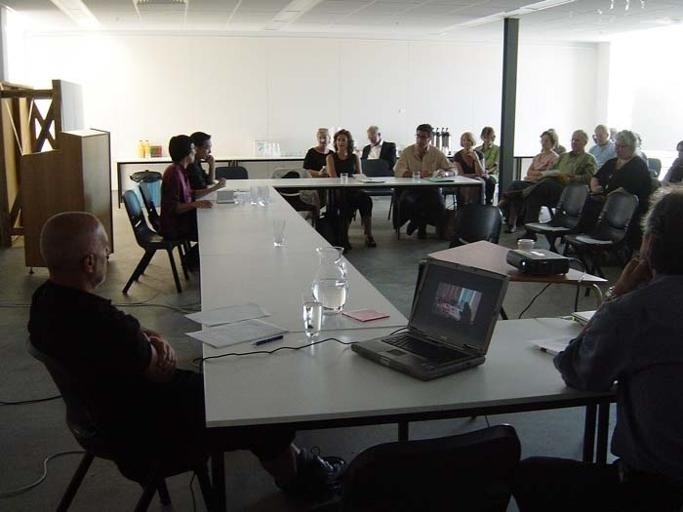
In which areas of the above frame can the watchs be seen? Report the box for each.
[603,286,620,301]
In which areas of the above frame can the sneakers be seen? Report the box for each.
[366,237,376,247]
[504,224,516,233]
[407,223,427,239]
[276,447,345,499]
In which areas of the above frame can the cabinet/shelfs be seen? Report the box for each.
[117,159,305,208]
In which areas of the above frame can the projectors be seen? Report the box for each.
[505,247,570,274]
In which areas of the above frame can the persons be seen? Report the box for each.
[662,141,682,187]
[326,129,377,250]
[304,128,337,207]
[186,132,216,190]
[159,135,226,278]
[498,125,660,265]
[453,127,501,207]
[394,124,452,241]
[28,210,347,507]
[362,126,397,189]
[511,189,682,512]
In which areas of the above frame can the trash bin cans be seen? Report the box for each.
[132,170,161,207]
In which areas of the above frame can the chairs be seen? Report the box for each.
[327,158,661,319]
[215,167,248,179]
[122,190,188,294]
[310,425,520,511]
[270,168,312,178]
[139,186,191,272]
[25,337,211,512]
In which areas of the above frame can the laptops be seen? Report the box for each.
[351,256,509,382]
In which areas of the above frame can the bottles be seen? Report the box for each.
[429,126,449,150]
[137,138,152,158]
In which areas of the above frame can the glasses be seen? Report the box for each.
[190,146,198,151]
[416,133,431,140]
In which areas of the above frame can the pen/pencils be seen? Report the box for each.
[252,334,284,347]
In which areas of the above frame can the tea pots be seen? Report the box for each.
[589,280,614,311]
[306,244,349,314]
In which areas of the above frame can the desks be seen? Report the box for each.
[194,175,618,464]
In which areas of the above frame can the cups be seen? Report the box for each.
[304,338,322,357]
[517,237,535,251]
[270,216,288,247]
[299,287,328,344]
[412,170,421,184]
[340,172,348,184]
[268,143,280,157]
[248,186,271,208]
